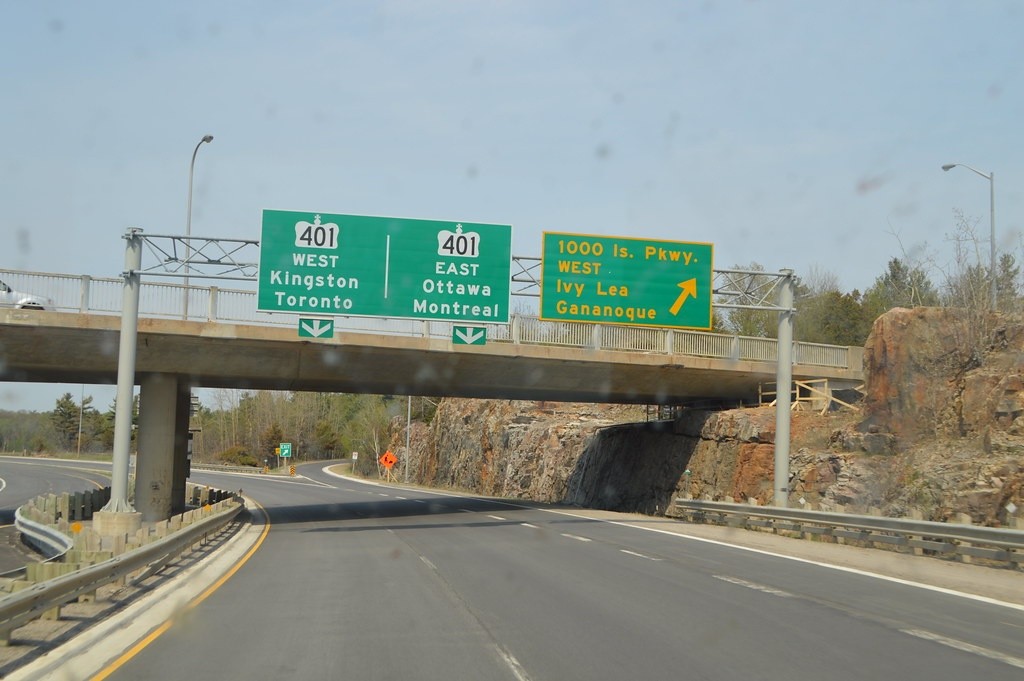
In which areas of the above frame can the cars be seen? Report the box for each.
[0,278,56,312]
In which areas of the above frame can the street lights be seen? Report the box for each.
[183,133,217,320]
[940,162,1002,313]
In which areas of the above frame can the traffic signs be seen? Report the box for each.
[540,229,712,331]
[280,443,292,457]
